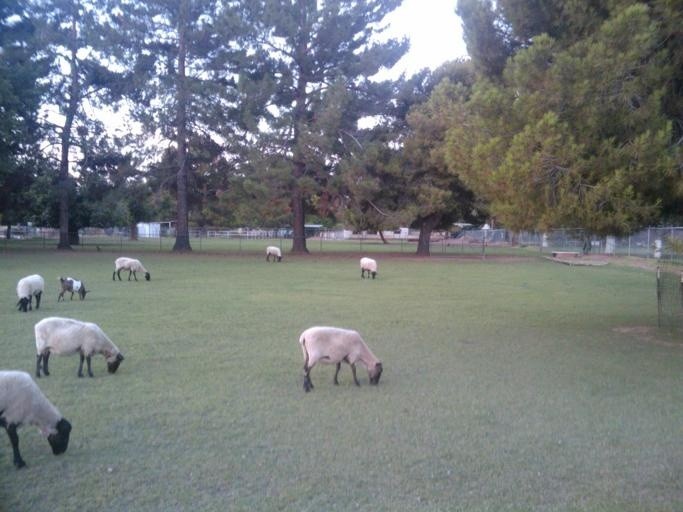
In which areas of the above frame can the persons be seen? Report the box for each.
[649,234,663,271]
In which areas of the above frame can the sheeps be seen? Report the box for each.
[0,370,72,469]
[56,277,90,302]
[16,274,44,312]
[299,326,384,393]
[34,316,125,378]
[360,257,378,279]
[264,246,282,262]
[113,256,150,281]
